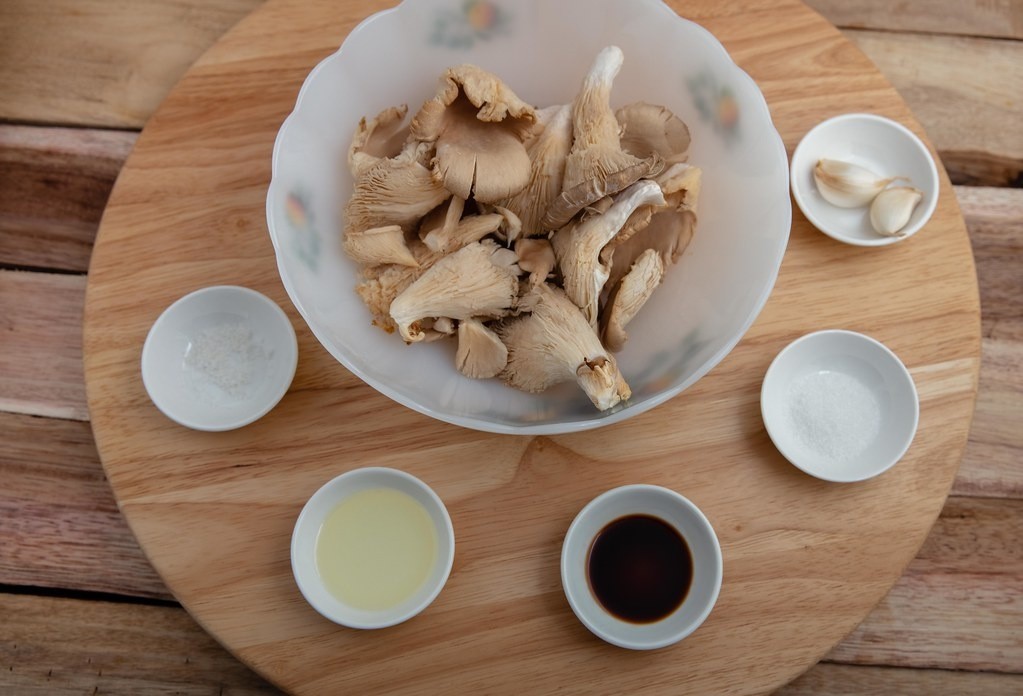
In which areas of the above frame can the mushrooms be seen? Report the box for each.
[341,46,700,409]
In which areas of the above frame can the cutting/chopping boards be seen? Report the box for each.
[83,0,986,695]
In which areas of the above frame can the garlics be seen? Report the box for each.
[814,159,922,234]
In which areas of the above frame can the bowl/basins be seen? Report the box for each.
[265,0,793,435]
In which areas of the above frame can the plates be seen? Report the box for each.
[560,483,723,650]
[761,327,920,483]
[289,466,454,630]
[140,285,299,433]
[789,114,940,249]
